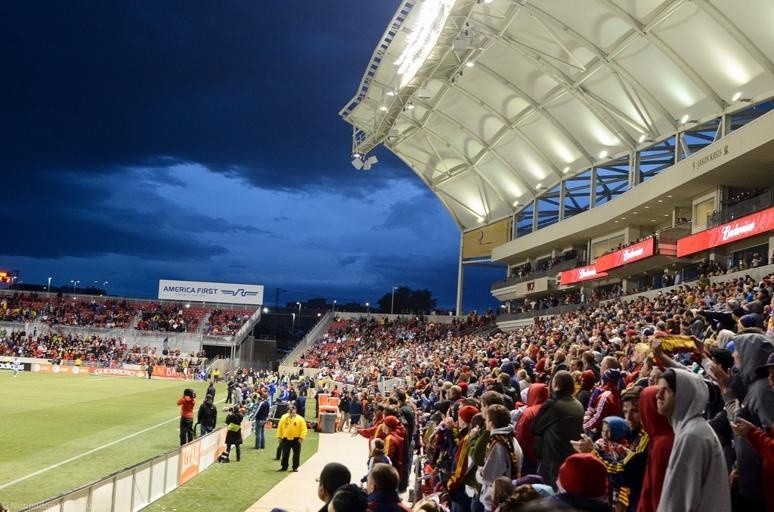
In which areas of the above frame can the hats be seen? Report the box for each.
[554,453,609,498]
[384,415,398,429]
[600,368,621,385]
[656,368,676,393]
[459,405,480,424]
[607,336,623,346]
[738,313,764,331]
[370,438,385,451]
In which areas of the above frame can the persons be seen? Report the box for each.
[504,187,774,283]
[0,291,258,383]
[177,272,774,511]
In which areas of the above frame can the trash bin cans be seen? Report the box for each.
[322,414,336,433]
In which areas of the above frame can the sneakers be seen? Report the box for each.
[253,446,264,449]
[278,467,287,471]
[237,457,241,462]
[293,468,298,472]
[273,457,280,460]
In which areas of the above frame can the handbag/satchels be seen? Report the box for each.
[227,422,241,432]
